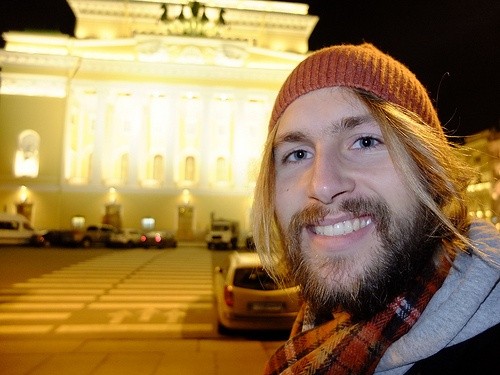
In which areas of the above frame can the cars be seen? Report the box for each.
[108,228,145,250]
[32,229,70,248]
[214,250,306,337]
[140,230,178,249]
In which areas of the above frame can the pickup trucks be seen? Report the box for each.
[62,222,118,249]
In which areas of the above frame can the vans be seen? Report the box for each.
[0,213,39,246]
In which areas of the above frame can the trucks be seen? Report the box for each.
[204,218,239,251]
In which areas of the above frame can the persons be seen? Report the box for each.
[251,44,500,375]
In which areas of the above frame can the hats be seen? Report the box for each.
[268,42,448,144]
